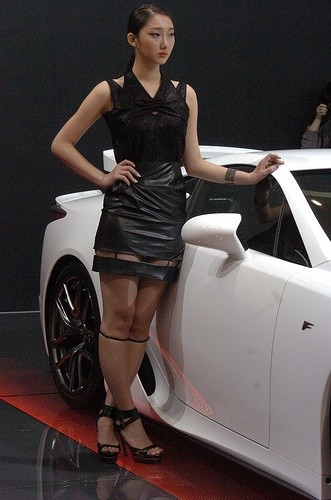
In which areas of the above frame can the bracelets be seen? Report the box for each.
[224,168,237,187]
[254,195,270,208]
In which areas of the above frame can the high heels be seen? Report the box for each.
[117,406,164,462]
[96,404,120,463]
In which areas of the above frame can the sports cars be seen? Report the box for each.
[37,144,331,500]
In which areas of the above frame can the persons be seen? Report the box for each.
[300,103,331,147]
[51,2,288,464]
[254,175,299,264]
